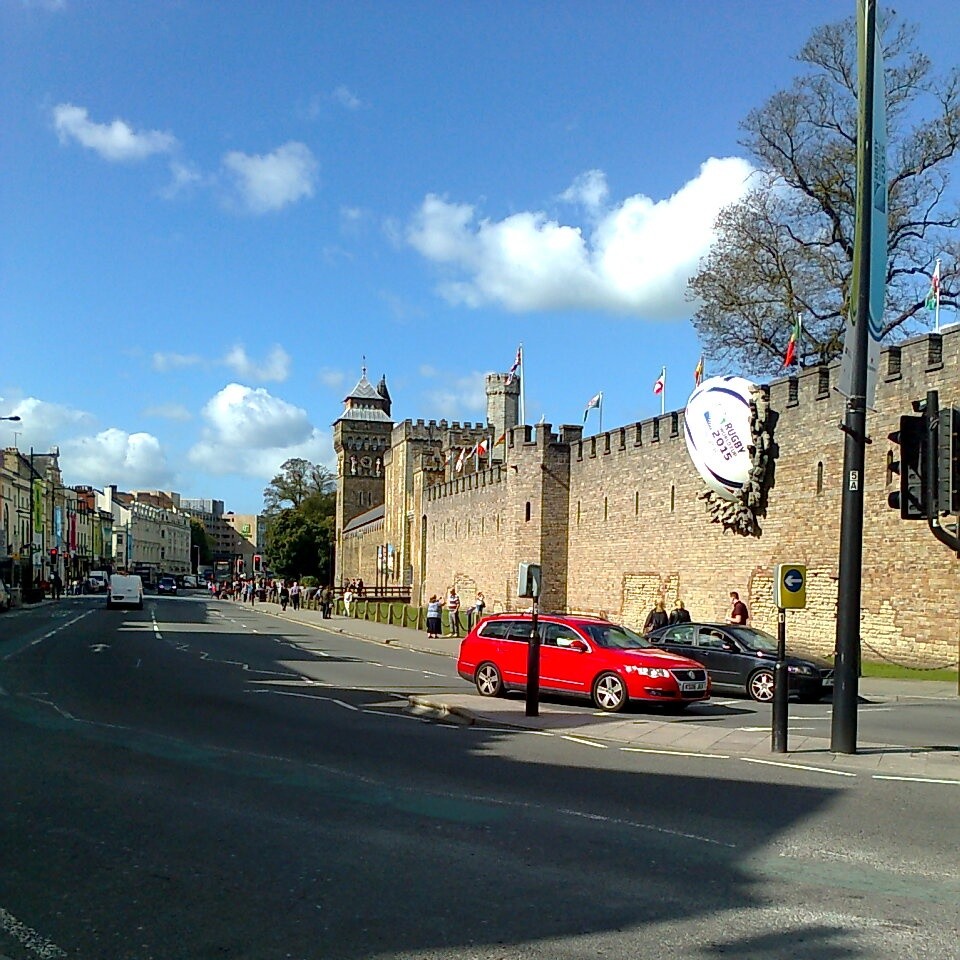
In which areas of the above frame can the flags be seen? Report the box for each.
[694,358,703,387]
[924,265,939,310]
[782,315,798,368]
[465,442,477,461]
[494,432,511,446]
[455,447,465,472]
[503,347,521,386]
[654,372,662,394]
[478,439,494,455]
[582,394,599,422]
[541,414,545,423]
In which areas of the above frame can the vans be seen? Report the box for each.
[88,570,108,592]
[107,573,145,606]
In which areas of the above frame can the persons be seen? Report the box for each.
[475,592,486,617]
[212,580,335,619]
[667,599,692,624]
[344,578,364,597]
[426,595,447,639]
[344,587,353,617]
[447,587,460,638]
[644,599,670,633]
[726,592,749,626]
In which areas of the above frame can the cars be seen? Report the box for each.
[456,611,710,712]
[644,619,837,704]
[158,577,178,594]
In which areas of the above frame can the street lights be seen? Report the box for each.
[26,446,58,598]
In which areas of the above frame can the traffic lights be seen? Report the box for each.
[50,549,57,564]
[238,560,244,574]
[253,555,261,571]
[887,416,927,518]
